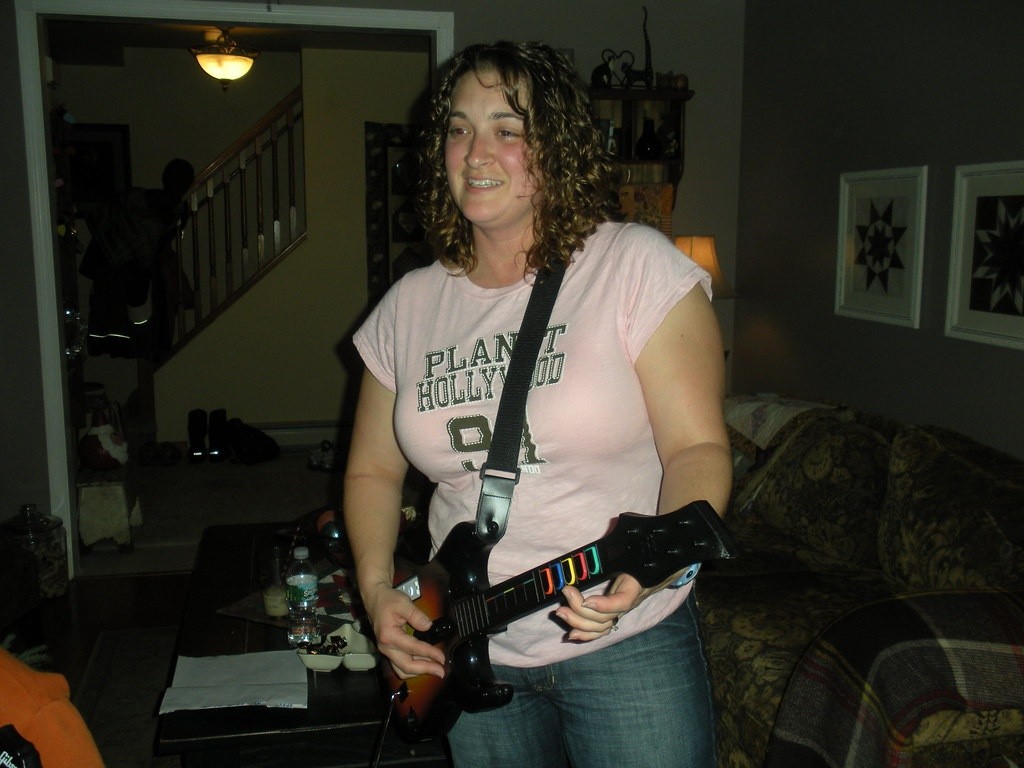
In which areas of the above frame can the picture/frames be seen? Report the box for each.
[944,160,1024,350]
[834,165,928,328]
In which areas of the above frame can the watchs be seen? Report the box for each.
[667,563,701,589]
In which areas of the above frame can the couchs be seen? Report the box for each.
[696,394,1024,768]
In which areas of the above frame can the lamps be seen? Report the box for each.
[674,235,723,296]
[189,26,256,93]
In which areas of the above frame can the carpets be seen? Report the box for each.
[73,626,181,768]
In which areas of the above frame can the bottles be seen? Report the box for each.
[285,548,319,648]
[0,503,69,595]
[261,547,289,621]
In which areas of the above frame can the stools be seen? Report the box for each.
[76,459,143,555]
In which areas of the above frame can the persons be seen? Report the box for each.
[343,39,733,768]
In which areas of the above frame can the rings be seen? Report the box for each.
[611,616,619,633]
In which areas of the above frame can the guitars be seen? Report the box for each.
[371,498,725,736]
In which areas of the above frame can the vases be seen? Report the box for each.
[635,101,661,159]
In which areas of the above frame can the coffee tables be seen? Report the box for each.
[155,521,454,768]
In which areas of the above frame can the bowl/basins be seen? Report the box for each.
[296,648,344,672]
[342,653,379,671]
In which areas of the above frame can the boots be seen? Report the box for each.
[187,409,208,464]
[208,408,232,464]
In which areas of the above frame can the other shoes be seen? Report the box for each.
[308,446,323,468]
[320,440,336,471]
